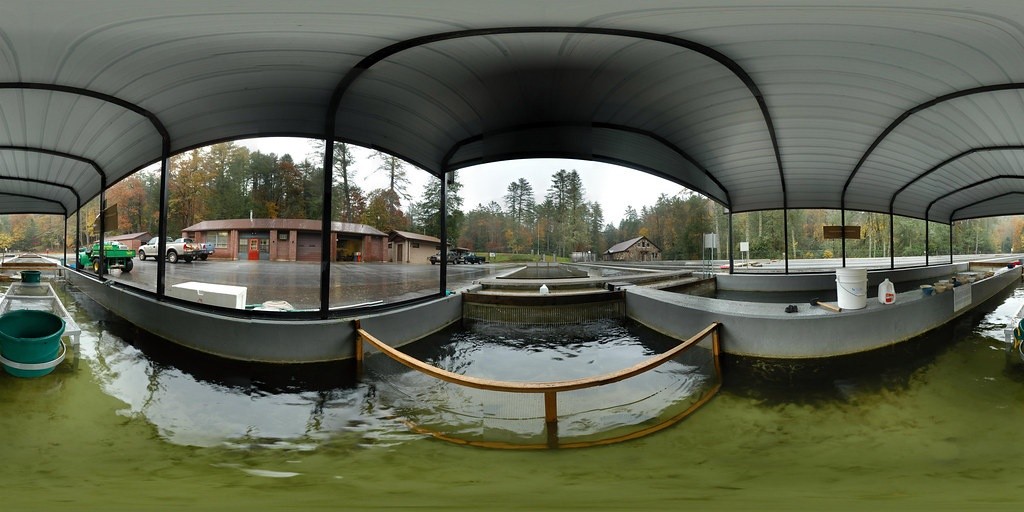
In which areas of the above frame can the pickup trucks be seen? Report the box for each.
[138,236,202,263]
[457,252,485,265]
[174,237,216,261]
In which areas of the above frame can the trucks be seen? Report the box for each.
[77,240,136,274]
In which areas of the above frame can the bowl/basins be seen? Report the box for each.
[1007,264,1013,268]
[920,271,994,295]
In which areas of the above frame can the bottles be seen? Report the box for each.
[878,278,896,304]
[540,284,548,294]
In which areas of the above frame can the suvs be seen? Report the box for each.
[429,251,459,265]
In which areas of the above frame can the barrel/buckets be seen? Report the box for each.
[21,271,41,283]
[835,268,868,309]
[0,309,66,378]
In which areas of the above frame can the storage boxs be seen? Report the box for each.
[171,281,247,309]
